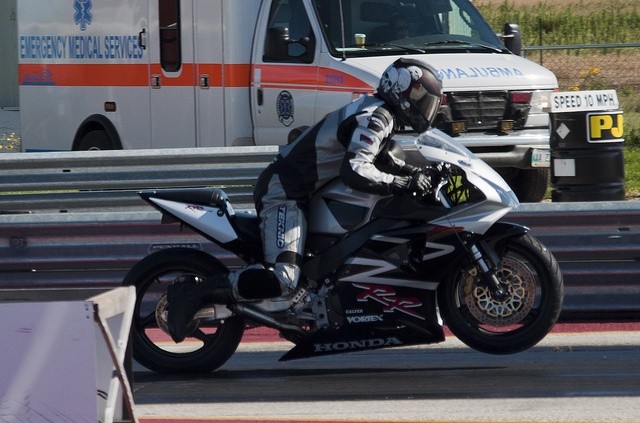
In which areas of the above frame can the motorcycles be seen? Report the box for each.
[121,125,566,385]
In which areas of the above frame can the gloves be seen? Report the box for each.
[400,164,435,176]
[393,174,431,196]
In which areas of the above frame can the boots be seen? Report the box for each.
[167,273,235,343]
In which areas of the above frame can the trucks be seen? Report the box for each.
[16,0,558,202]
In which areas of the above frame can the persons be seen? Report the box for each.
[166,56,445,344]
[387,13,410,40]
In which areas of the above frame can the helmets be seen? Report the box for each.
[376,58,443,134]
